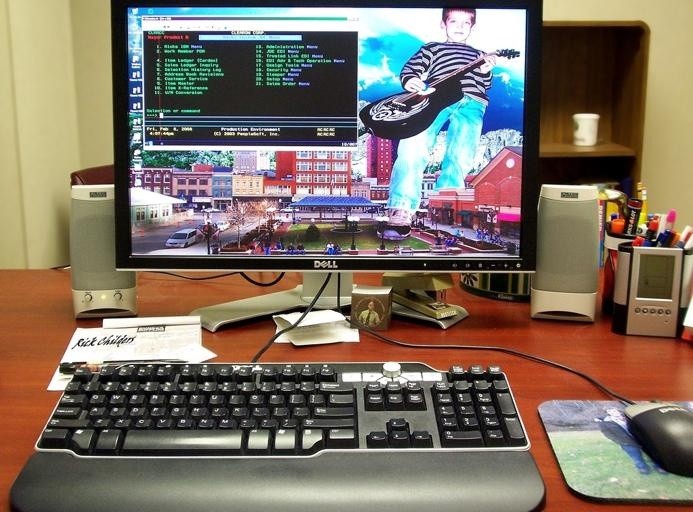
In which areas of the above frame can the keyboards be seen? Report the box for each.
[8,360,548,512]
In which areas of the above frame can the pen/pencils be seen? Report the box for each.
[611,181,693,249]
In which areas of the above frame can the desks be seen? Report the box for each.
[1,268,693,511]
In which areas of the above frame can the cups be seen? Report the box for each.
[571,113,598,147]
[603,232,692,340]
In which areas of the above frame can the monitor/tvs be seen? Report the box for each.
[110,0,544,332]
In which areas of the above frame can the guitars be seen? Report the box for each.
[360,48,520,139]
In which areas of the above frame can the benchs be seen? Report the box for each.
[388,246,463,255]
[270,248,349,255]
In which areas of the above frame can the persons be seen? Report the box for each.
[375,9,495,241]
[434,226,507,249]
[250,235,339,257]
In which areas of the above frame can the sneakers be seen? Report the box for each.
[374,206,420,242]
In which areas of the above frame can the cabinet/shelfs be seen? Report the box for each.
[537,18,651,202]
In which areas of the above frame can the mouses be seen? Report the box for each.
[624,397,693,477]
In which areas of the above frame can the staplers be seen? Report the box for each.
[380,273,458,319]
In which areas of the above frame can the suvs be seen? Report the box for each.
[279,207,299,213]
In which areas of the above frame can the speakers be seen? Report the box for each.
[530,183,602,322]
[69,184,136,320]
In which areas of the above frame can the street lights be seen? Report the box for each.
[376,216,388,249]
[265,207,275,240]
[419,209,427,230]
[345,216,360,250]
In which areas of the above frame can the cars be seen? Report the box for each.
[165,216,244,249]
